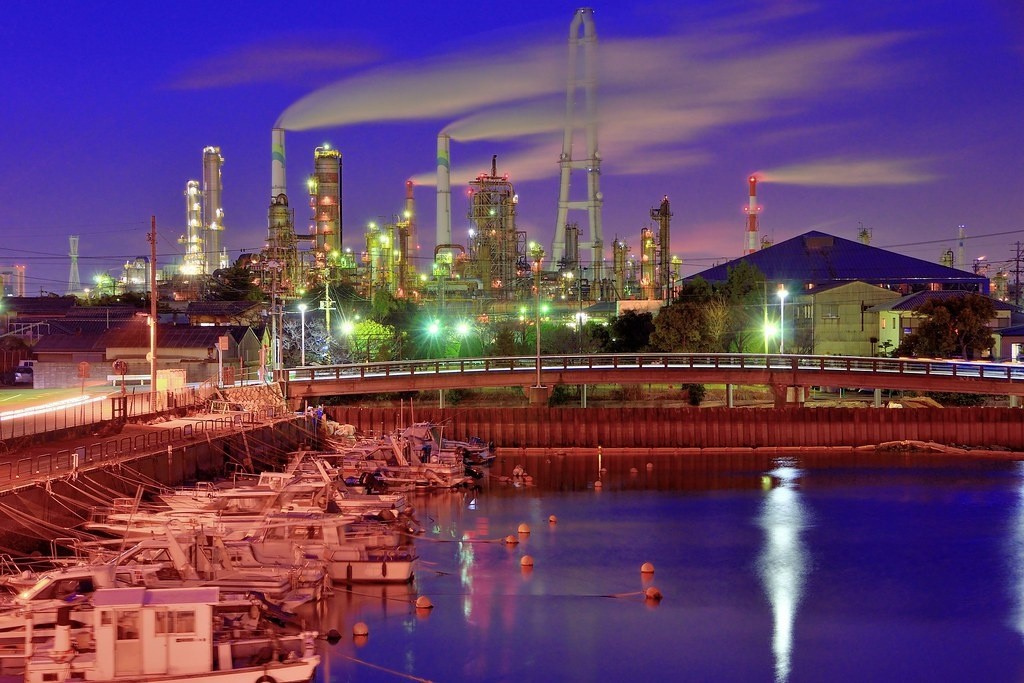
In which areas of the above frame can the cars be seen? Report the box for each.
[1,366,33,386]
[858,389,874,394]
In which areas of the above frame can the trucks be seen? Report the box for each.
[19,360,37,371]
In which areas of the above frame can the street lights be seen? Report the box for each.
[135,312,156,411]
[777,289,788,354]
[297,304,308,369]
[84,289,89,300]
[532,249,544,387]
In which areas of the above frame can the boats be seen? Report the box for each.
[0,422,497,683]
